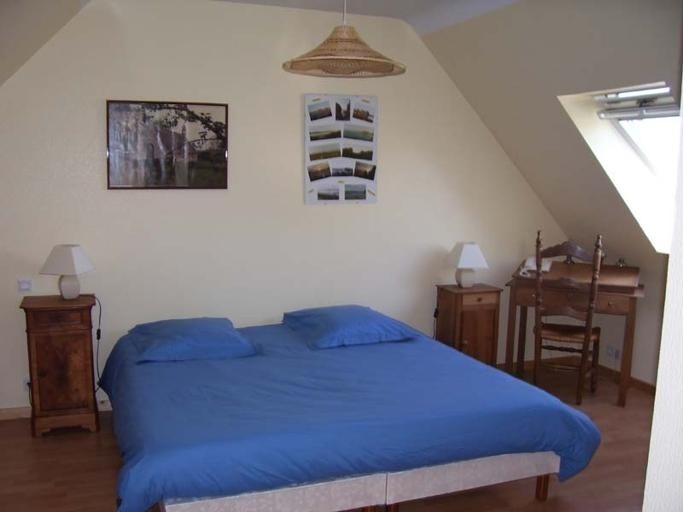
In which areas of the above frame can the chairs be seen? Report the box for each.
[529,230,603,406]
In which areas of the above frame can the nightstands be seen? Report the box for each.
[433,283,504,370]
[18,292,101,439]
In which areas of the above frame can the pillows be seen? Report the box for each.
[125,317,256,366]
[282,305,423,352]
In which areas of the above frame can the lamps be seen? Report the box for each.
[39,242,95,300]
[281,0,407,78]
[445,242,490,288]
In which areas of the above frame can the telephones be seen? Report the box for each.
[524,256,553,272]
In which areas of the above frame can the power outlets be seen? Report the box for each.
[24,375,30,392]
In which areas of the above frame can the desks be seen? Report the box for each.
[504,260,646,407]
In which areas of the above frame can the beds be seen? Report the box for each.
[102,315,601,512]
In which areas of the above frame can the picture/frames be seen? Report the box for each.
[105,99,229,190]
